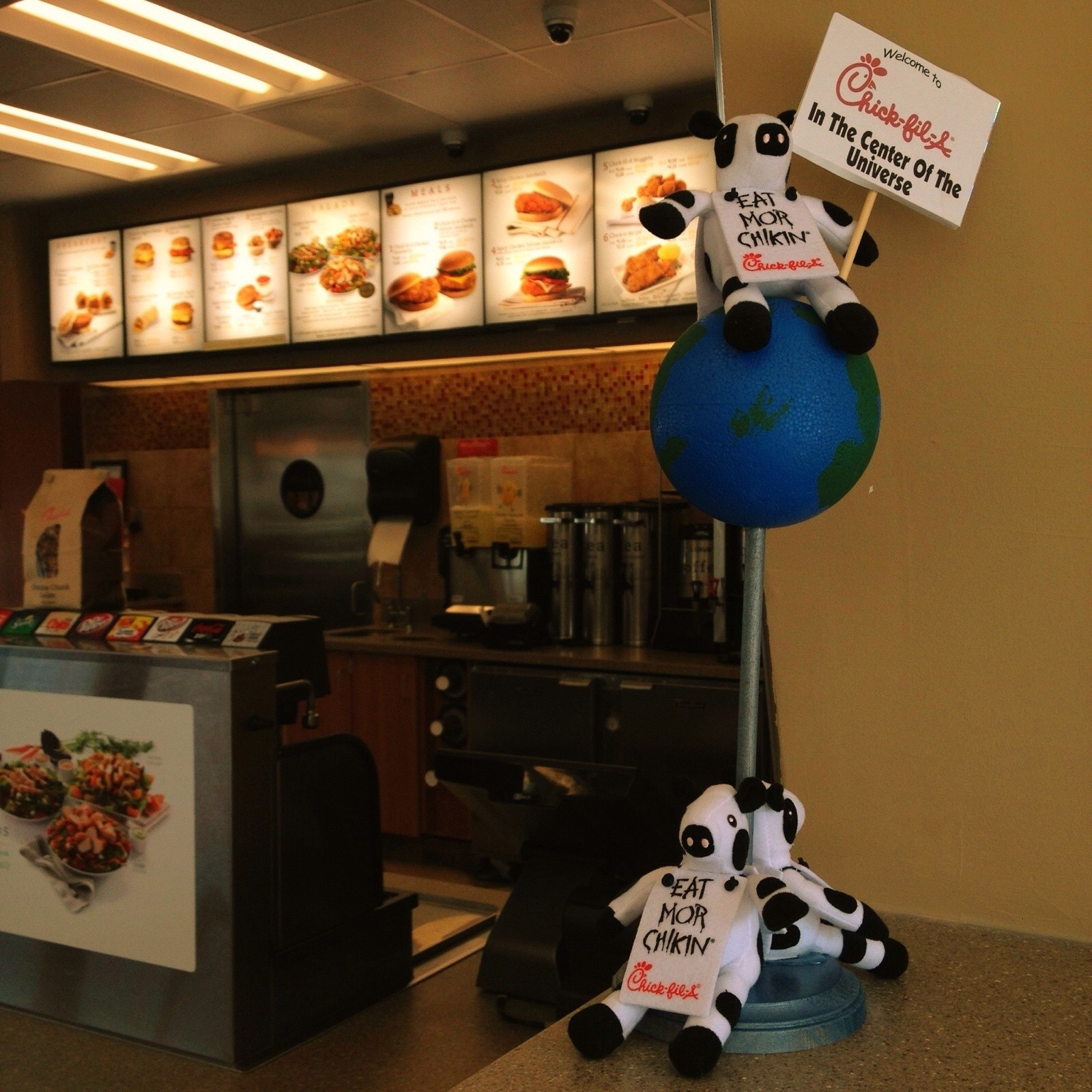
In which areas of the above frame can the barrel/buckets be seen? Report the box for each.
[574,499,622,649]
[614,496,691,646]
[542,502,582,647]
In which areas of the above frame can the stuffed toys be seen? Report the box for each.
[640,108,882,356]
[750,777,909,982]
[567,768,769,1081]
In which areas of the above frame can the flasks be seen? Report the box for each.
[676,523,718,613]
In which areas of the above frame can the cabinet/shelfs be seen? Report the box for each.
[332,651,425,835]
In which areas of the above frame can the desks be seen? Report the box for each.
[461,911,1092,1091]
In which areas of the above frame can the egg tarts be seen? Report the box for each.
[256,275,271,286]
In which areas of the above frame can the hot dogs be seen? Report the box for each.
[134,306,158,331]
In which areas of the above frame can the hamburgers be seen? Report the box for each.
[171,301,193,332]
[132,242,155,269]
[387,250,478,311]
[515,180,572,222]
[212,231,237,259]
[168,236,194,264]
[521,256,572,302]
[57,290,117,337]
